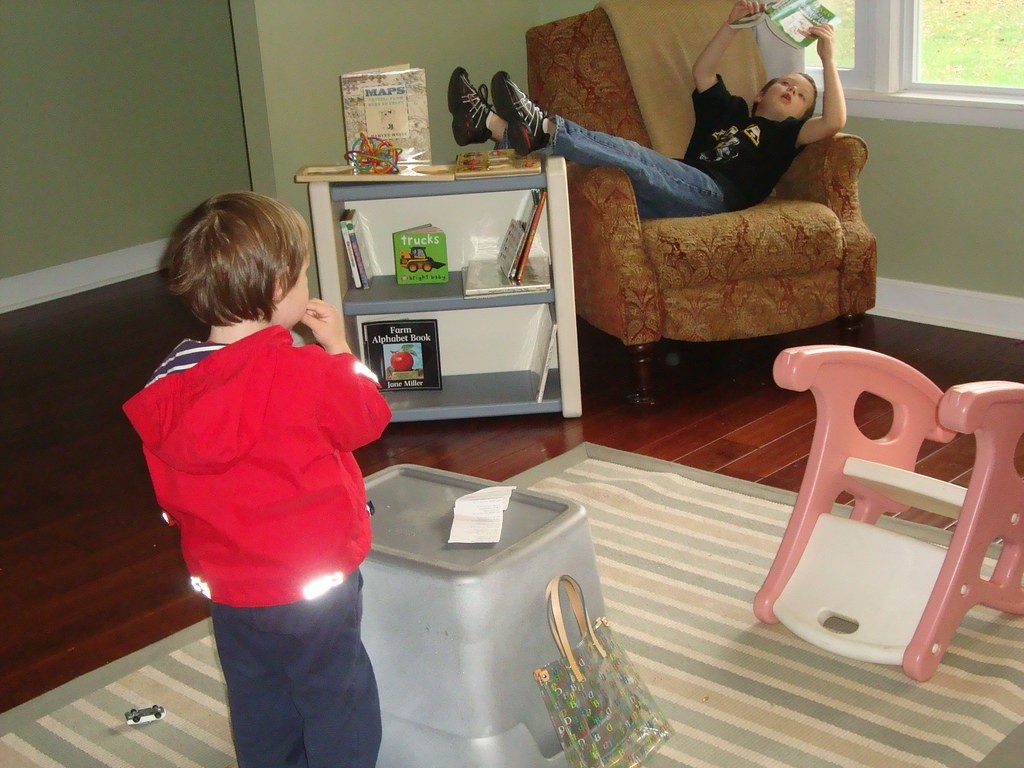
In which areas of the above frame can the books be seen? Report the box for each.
[339,208,373,289]
[340,64,432,166]
[728,0,841,49]
[530,303,557,403]
[462,189,552,300]
[361,318,442,392]
[393,224,449,284]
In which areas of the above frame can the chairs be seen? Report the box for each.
[754,343,1024,682]
[525,7,877,404]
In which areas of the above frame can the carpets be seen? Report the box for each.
[0,439,1024,767]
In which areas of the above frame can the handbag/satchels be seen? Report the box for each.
[535,574,674,768]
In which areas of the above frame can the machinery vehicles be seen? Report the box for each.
[400,246,446,272]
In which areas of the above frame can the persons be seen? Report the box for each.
[123,190,392,768]
[448,0,846,218]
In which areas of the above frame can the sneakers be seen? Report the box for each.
[448,66,493,146]
[491,70,551,156]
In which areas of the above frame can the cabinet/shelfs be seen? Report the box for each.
[309,155,583,423]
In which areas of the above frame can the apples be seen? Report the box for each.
[389,349,415,371]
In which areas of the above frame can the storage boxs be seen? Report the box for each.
[356,463,612,768]
